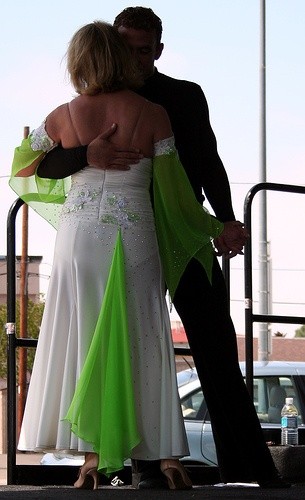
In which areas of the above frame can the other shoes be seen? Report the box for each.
[138,470,164,489]
[256,470,292,488]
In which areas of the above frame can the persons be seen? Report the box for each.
[37,6,293,487]
[7,21,248,489]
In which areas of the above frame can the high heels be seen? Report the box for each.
[74,454,99,490]
[160,457,193,490]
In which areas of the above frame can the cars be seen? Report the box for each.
[39,360,305,466]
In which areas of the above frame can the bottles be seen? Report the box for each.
[281,397,298,446]
